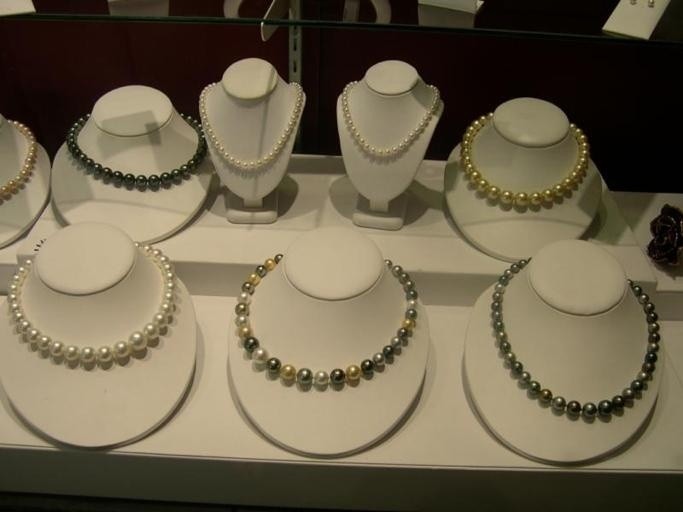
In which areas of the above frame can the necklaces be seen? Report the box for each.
[460,112,590,207]
[199,81,304,169]
[7,241,178,365]
[0,118,38,203]
[490,255,661,418]
[67,113,206,187]
[341,80,441,157]
[233,254,419,387]
